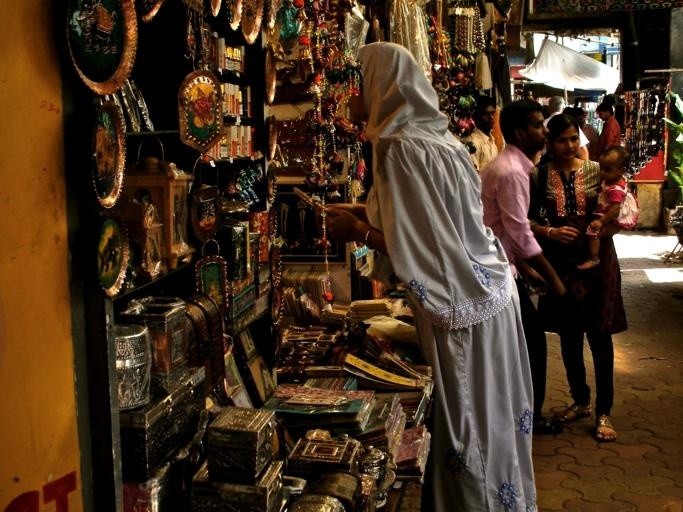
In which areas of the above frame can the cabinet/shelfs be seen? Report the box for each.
[58,0,426,512]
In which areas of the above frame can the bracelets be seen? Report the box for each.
[546,226,553,238]
[363,228,371,247]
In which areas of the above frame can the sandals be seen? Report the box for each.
[534,418,563,436]
[596,416,616,444]
[559,404,592,422]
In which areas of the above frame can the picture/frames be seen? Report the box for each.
[276,181,347,265]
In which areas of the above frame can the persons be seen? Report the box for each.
[535,96,621,152]
[320,42,536,510]
[578,146,628,269]
[530,115,628,442]
[475,99,566,436]
[462,96,498,170]
